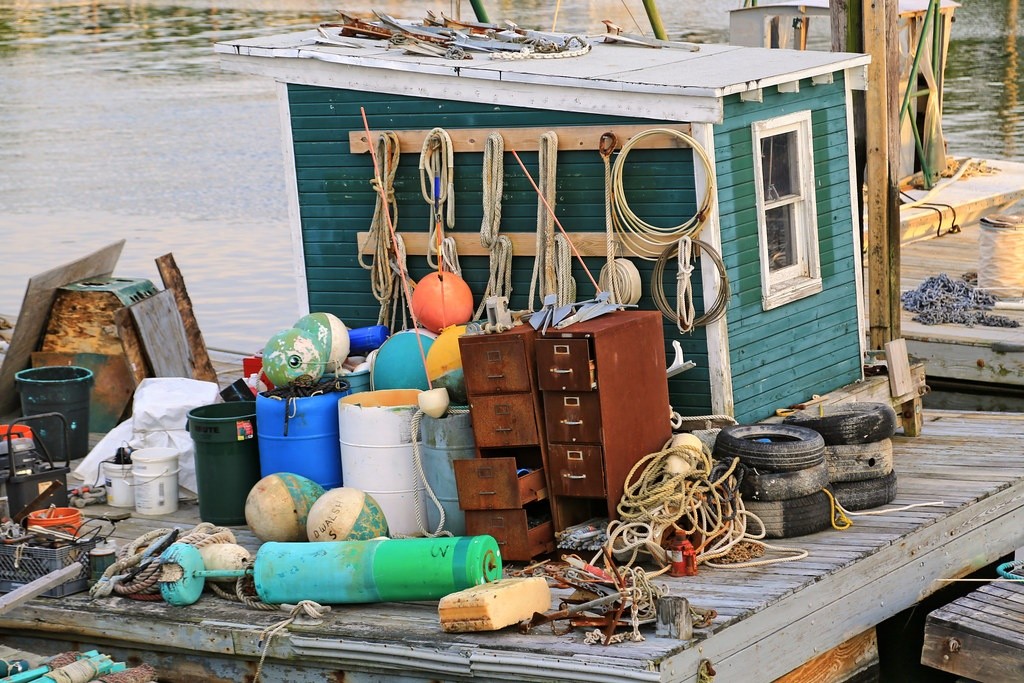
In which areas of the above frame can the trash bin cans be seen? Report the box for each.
[184,401,261,527]
[15,365,94,462]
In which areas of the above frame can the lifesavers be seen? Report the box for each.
[709,402,899,538]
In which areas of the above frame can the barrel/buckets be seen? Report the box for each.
[102,441,134,507]
[122,447,183,515]
[250,374,484,538]
[27,508,85,539]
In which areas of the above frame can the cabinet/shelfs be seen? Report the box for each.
[453,311,672,565]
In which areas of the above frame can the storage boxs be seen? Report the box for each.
[0,540,96,599]
